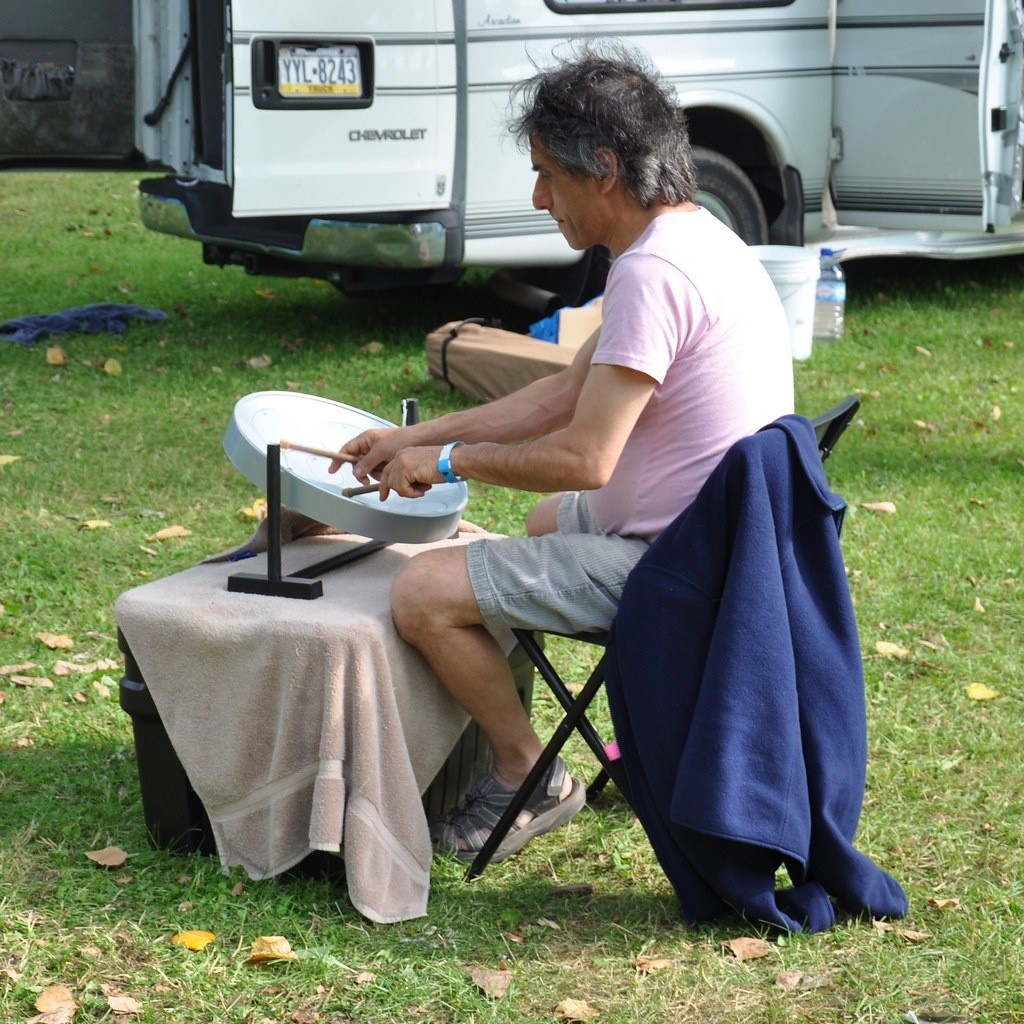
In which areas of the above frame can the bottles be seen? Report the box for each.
[812,248,847,339]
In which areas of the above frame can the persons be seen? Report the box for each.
[328,30,798,863]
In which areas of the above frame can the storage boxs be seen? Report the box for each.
[117,518,546,890]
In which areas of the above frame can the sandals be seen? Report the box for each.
[431,753,586,863]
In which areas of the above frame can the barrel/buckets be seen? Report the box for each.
[748,245,819,360]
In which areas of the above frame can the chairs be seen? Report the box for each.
[461,396,861,883]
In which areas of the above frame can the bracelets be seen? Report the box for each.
[433,439,466,484]
